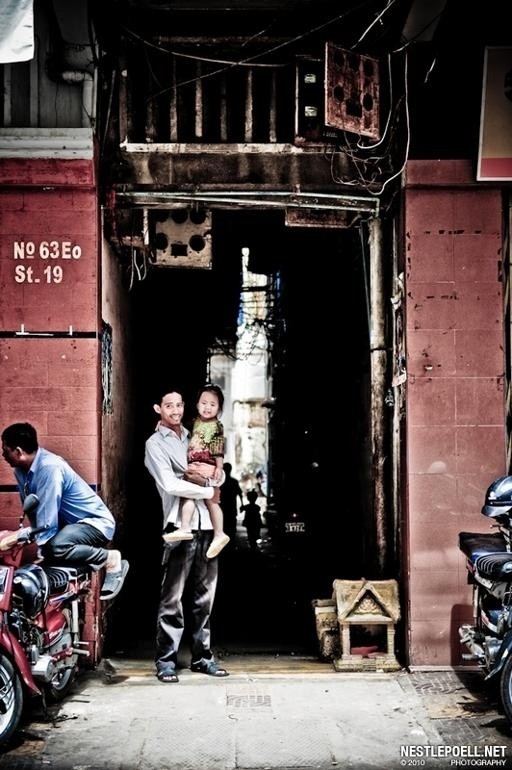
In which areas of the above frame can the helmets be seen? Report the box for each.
[12,564,49,619]
[481,475,512,518]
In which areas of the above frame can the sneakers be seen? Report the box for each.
[206,532,230,559]
[162,527,194,543]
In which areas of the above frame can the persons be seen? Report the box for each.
[219,463,268,555]
[162,386,231,559]
[144,389,229,682]
[1,423,129,599]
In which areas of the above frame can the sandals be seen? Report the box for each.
[156,661,178,682]
[191,656,230,677]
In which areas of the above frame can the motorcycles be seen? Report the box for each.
[454,474,511,721]
[1,494,92,753]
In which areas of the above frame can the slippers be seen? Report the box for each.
[99,559,130,600]
